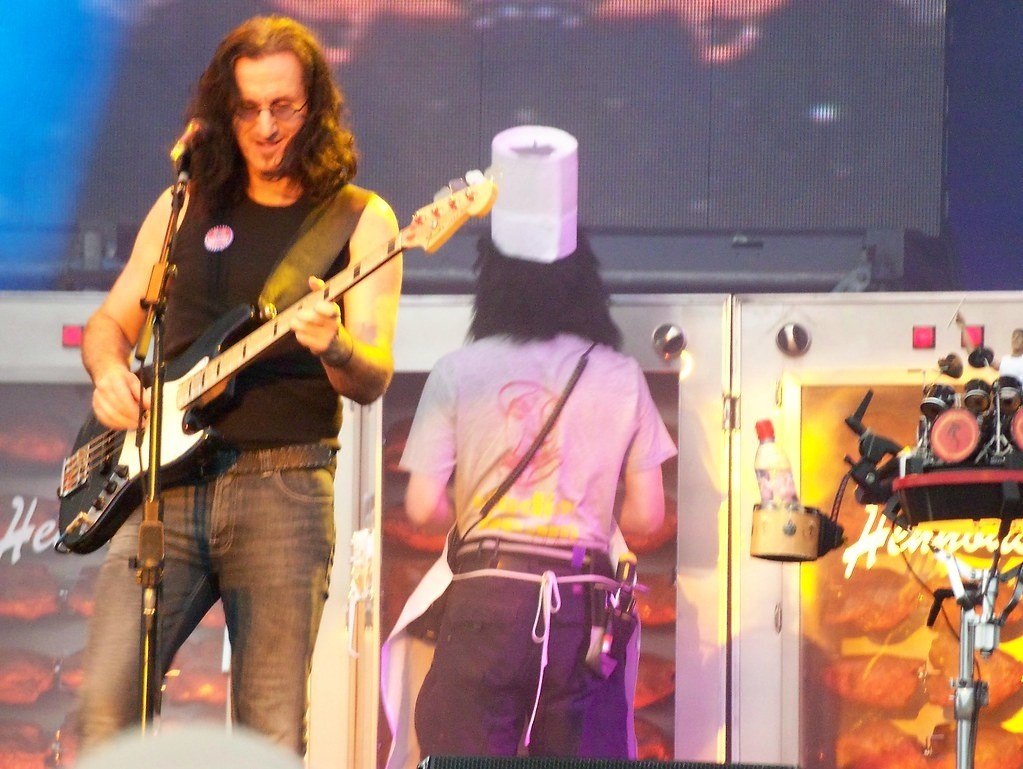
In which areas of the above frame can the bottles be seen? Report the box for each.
[755,419,799,513]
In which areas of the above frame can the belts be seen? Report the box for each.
[456,541,614,578]
[156,443,333,485]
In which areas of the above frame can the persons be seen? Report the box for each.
[83,13,402,765]
[401,126,678,759]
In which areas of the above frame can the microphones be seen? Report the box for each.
[169,115,212,163]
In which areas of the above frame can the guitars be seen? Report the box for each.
[57,165,502,555]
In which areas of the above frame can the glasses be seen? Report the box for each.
[231,95,312,119]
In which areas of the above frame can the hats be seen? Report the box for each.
[490,124,578,264]
